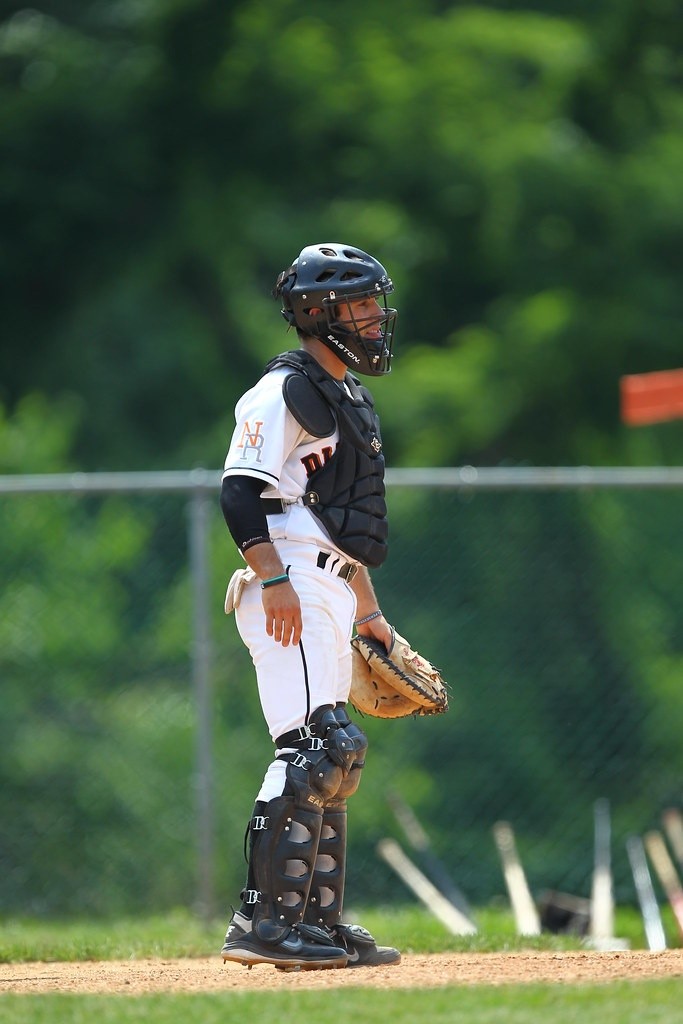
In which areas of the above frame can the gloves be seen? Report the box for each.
[224,568,256,614]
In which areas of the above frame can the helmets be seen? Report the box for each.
[276,243,392,376]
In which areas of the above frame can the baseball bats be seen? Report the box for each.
[374,794,683,951]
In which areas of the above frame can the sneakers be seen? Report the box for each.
[274,923,401,969]
[220,904,347,970]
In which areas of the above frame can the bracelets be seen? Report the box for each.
[260,574,290,589]
[352,606,383,628]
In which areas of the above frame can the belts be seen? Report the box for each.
[316,551,359,585]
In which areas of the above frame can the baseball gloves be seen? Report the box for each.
[346,621,455,722]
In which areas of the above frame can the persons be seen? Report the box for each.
[215,239,403,971]
